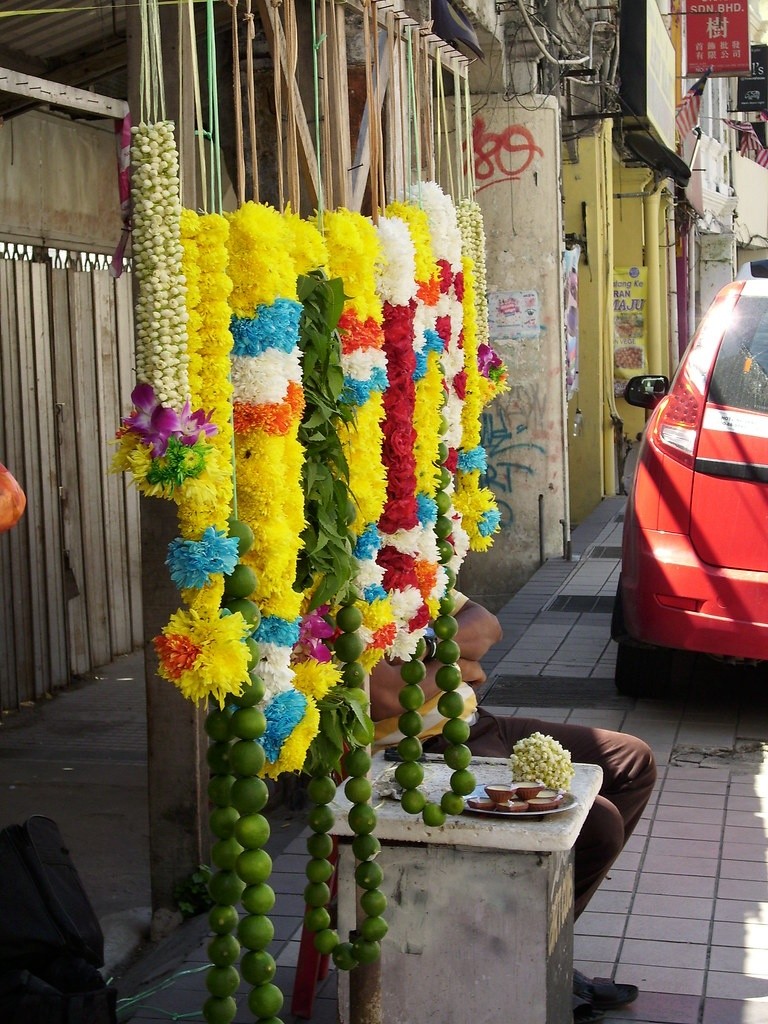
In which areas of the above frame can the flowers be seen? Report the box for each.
[111,183,510,780]
[509,732,572,788]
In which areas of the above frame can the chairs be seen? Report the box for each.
[291,744,353,1023]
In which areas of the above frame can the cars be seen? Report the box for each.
[612,259,768,701]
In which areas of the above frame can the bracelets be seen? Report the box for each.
[447,661,461,672]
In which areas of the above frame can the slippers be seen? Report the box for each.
[573,969,638,1009]
[572,994,606,1024]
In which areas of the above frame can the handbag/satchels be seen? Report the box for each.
[0,816,117,1024]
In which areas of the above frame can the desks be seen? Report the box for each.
[319,751,605,1024]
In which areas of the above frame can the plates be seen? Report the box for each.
[461,783,579,815]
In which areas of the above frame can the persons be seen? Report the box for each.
[352,584,659,1024]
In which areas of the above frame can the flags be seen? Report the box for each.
[722,112,768,170]
[673,64,717,145]
